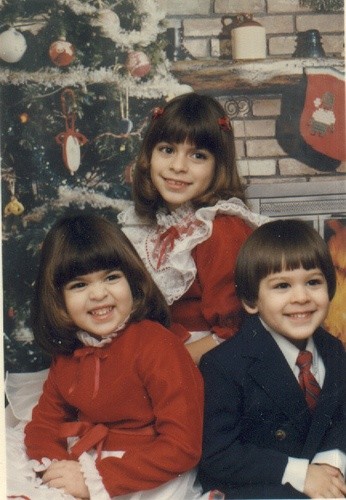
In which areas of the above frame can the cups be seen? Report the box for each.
[231,14,266,59]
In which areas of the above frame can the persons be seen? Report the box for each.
[198,219,346,500]
[116,94,273,364]
[1,214,202,500]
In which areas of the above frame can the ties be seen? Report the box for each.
[295,351,321,413]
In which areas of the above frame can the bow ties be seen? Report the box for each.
[151,227,183,270]
[73,346,109,399]
